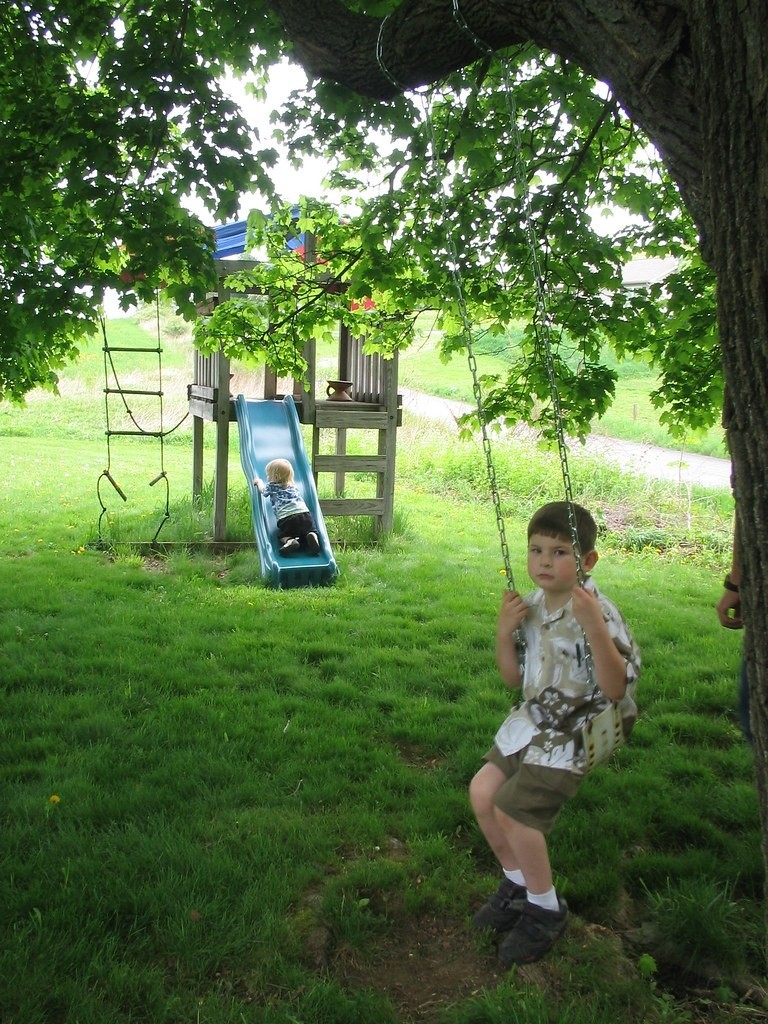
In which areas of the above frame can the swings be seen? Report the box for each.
[418,46,629,773]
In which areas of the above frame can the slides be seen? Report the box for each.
[234,389,340,589]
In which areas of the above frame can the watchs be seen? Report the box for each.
[723,575,739,591]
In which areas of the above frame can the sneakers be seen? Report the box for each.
[307,532,320,555]
[472,873,528,934]
[499,892,571,964]
[279,537,300,556]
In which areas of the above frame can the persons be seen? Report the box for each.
[716,474,752,746]
[254,459,320,556]
[470,501,641,967]
